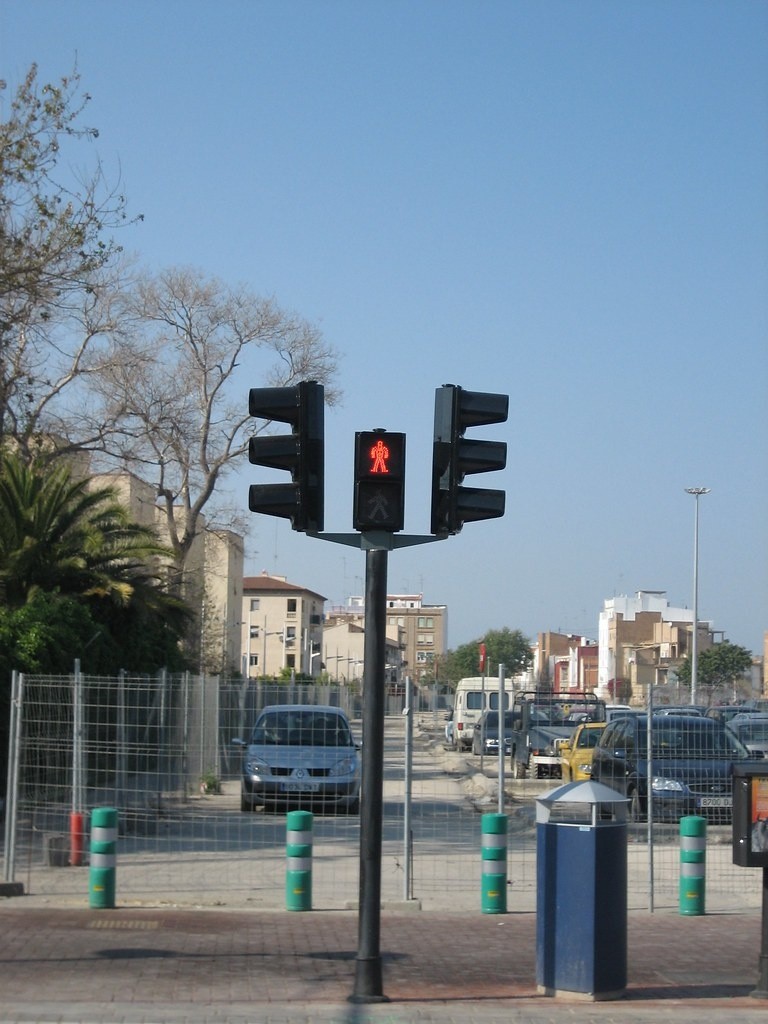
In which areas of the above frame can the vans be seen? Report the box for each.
[447,676,516,753]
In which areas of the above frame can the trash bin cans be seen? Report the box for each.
[731,759,768,866]
[534,780,630,1001]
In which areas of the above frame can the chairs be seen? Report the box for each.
[683,732,714,754]
[584,734,599,747]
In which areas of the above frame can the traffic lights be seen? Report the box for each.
[247,379,326,535]
[352,431,406,532]
[431,383,509,534]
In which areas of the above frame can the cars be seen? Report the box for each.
[589,714,765,824]
[471,710,523,756]
[558,722,609,785]
[602,704,768,759]
[232,704,360,816]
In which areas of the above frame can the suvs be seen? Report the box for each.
[511,692,607,779]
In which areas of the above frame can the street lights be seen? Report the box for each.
[684,486,711,706]
